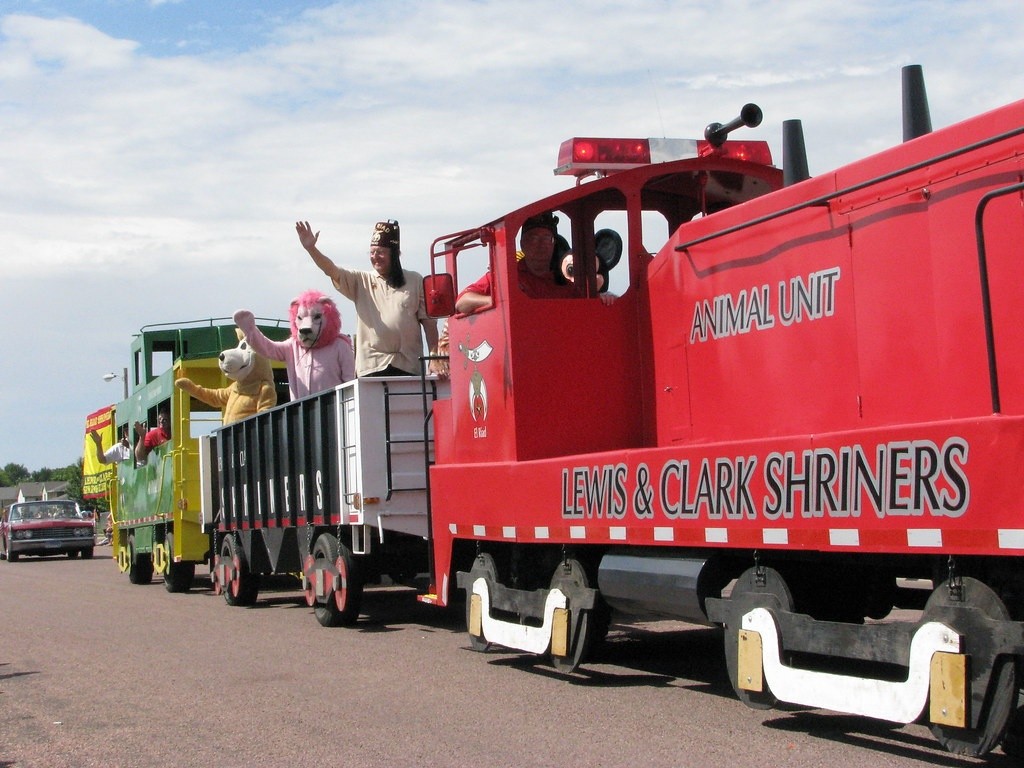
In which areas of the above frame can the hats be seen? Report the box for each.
[371,220,405,288]
[522,211,559,235]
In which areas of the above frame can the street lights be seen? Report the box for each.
[103,367,128,398]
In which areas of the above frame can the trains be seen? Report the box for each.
[101,95,1024,763]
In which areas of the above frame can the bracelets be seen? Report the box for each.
[429,352,438,357]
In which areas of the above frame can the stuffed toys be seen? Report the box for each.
[233,289,355,402]
[175,329,278,426]
[554,228,623,294]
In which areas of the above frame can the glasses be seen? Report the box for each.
[524,234,555,244]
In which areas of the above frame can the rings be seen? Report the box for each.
[442,369,445,372]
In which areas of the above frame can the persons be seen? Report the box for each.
[293,218,449,382]
[133,408,171,461]
[89,425,130,464]
[455,211,616,313]
[12,505,114,546]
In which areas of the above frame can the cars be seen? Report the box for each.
[0,500,96,560]
